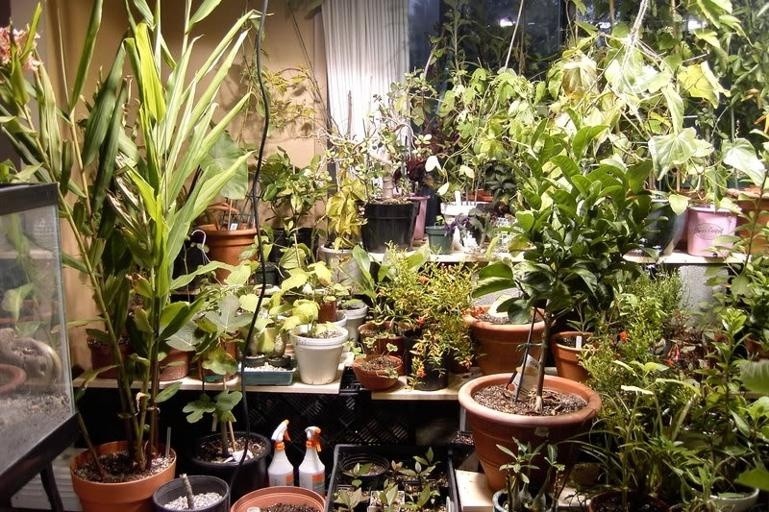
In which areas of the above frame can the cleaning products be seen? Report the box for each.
[267,419,295,486]
[298,426,325,498]
